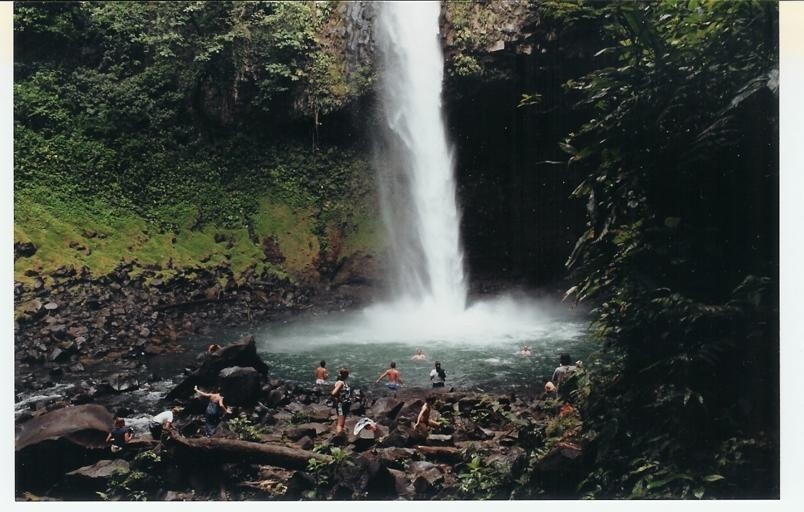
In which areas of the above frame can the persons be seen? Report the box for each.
[105,416,133,453]
[429,360,445,387]
[313,359,328,387]
[331,367,349,432]
[413,394,440,429]
[374,361,404,390]
[544,352,575,402]
[192,383,232,434]
[409,347,424,360]
[146,405,179,440]
